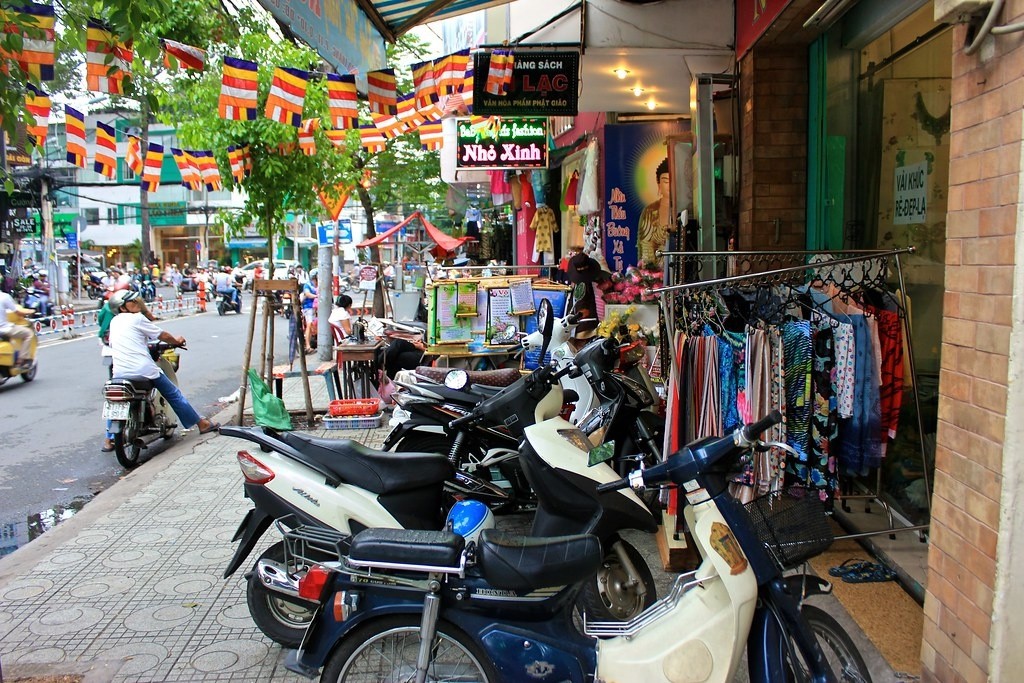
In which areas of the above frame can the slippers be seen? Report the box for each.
[200,421,221,434]
[829,558,876,577]
[101,444,115,451]
[842,564,898,583]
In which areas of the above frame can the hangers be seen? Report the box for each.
[543,194,548,209]
[675,257,906,337]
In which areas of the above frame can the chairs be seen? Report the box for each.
[329,322,345,370]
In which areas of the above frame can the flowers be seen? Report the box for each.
[597,305,659,346]
[596,260,665,305]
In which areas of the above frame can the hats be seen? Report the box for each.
[225,266,233,271]
[567,252,601,283]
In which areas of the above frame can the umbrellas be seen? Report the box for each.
[288,302,298,370]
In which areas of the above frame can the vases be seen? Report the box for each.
[604,303,659,328]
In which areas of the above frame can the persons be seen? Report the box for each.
[0,287,36,369]
[98,274,157,346]
[0,255,52,318]
[83,257,264,305]
[287,261,367,355]
[101,289,221,452]
[433,258,471,279]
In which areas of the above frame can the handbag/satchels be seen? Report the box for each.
[378,370,396,404]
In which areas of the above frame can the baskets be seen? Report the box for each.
[162,353,180,372]
[321,408,384,429]
[329,398,380,415]
[743,486,834,571]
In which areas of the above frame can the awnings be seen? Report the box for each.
[256,0,520,76]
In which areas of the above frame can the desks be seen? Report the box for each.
[332,336,394,400]
[424,347,518,369]
[273,361,343,401]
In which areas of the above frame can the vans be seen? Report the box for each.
[241,259,300,280]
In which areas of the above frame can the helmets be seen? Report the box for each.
[110,265,115,269]
[220,266,226,272]
[106,269,111,274]
[108,290,140,315]
[117,262,122,266]
[114,274,129,291]
[446,499,496,546]
[309,268,318,280]
[38,270,47,276]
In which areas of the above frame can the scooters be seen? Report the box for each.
[87,279,156,303]
[101,339,188,470]
[99,315,164,378]
[0,304,42,385]
[20,283,56,326]
[218,297,659,650]
[153,276,292,320]
[382,283,665,525]
[286,313,334,358]
[274,409,873,683]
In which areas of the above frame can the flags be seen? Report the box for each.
[0,0,515,193]
